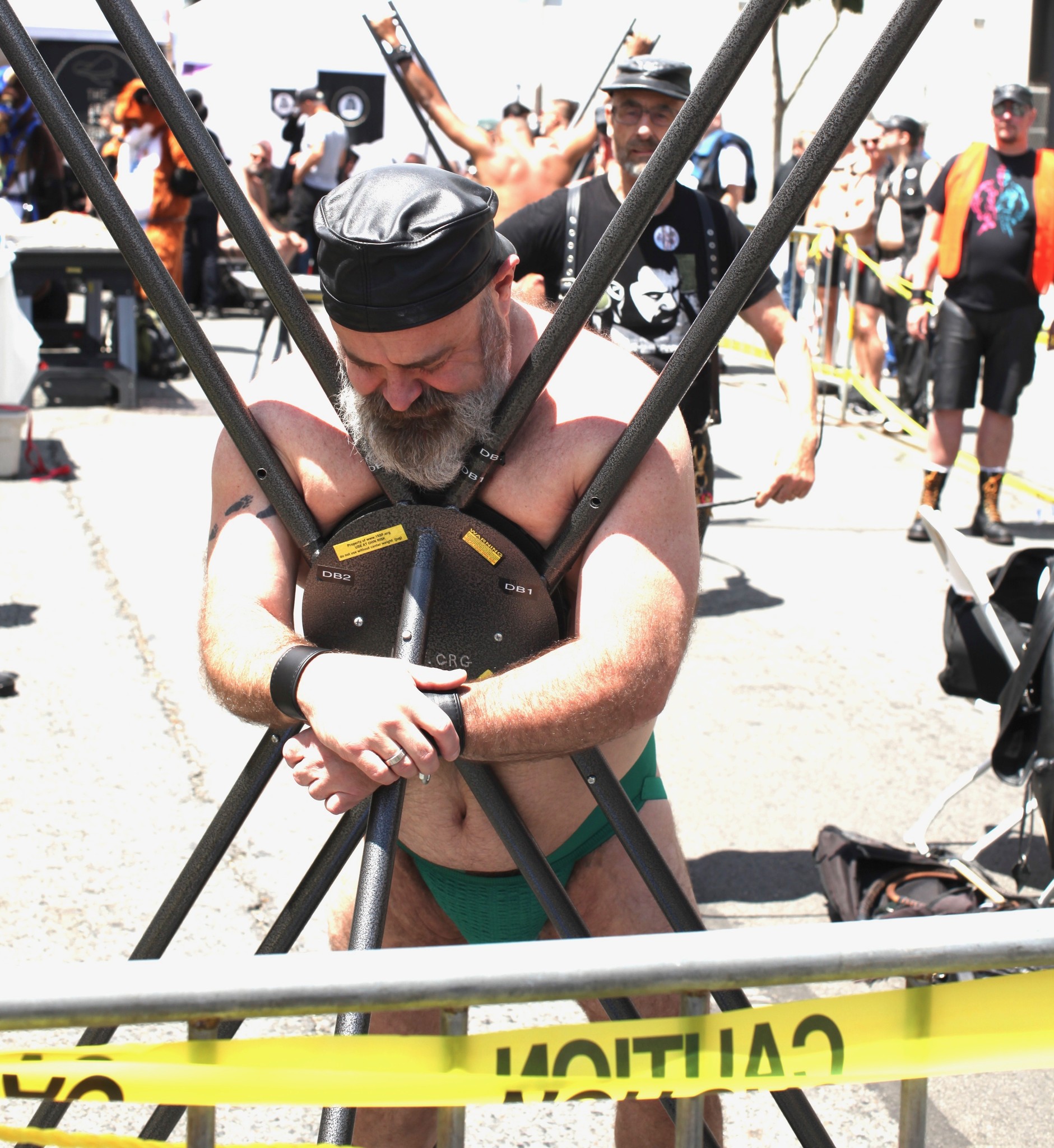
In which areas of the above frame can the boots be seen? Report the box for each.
[907,468,948,542]
[971,470,1014,545]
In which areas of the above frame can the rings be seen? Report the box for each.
[384,747,407,767]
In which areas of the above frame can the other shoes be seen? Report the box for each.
[191,303,225,320]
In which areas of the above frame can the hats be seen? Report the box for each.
[312,163,518,332]
[876,115,919,138]
[600,54,692,100]
[293,89,326,106]
[993,84,1033,107]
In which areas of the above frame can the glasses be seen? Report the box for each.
[610,104,680,127]
[861,138,879,145]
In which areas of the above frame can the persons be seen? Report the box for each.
[1,65,613,324]
[687,108,757,219]
[904,83,1054,546]
[491,56,825,547]
[369,17,654,231]
[769,114,944,436]
[200,162,724,1148]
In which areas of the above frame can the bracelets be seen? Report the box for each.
[271,645,332,721]
[417,688,465,757]
[910,290,925,298]
